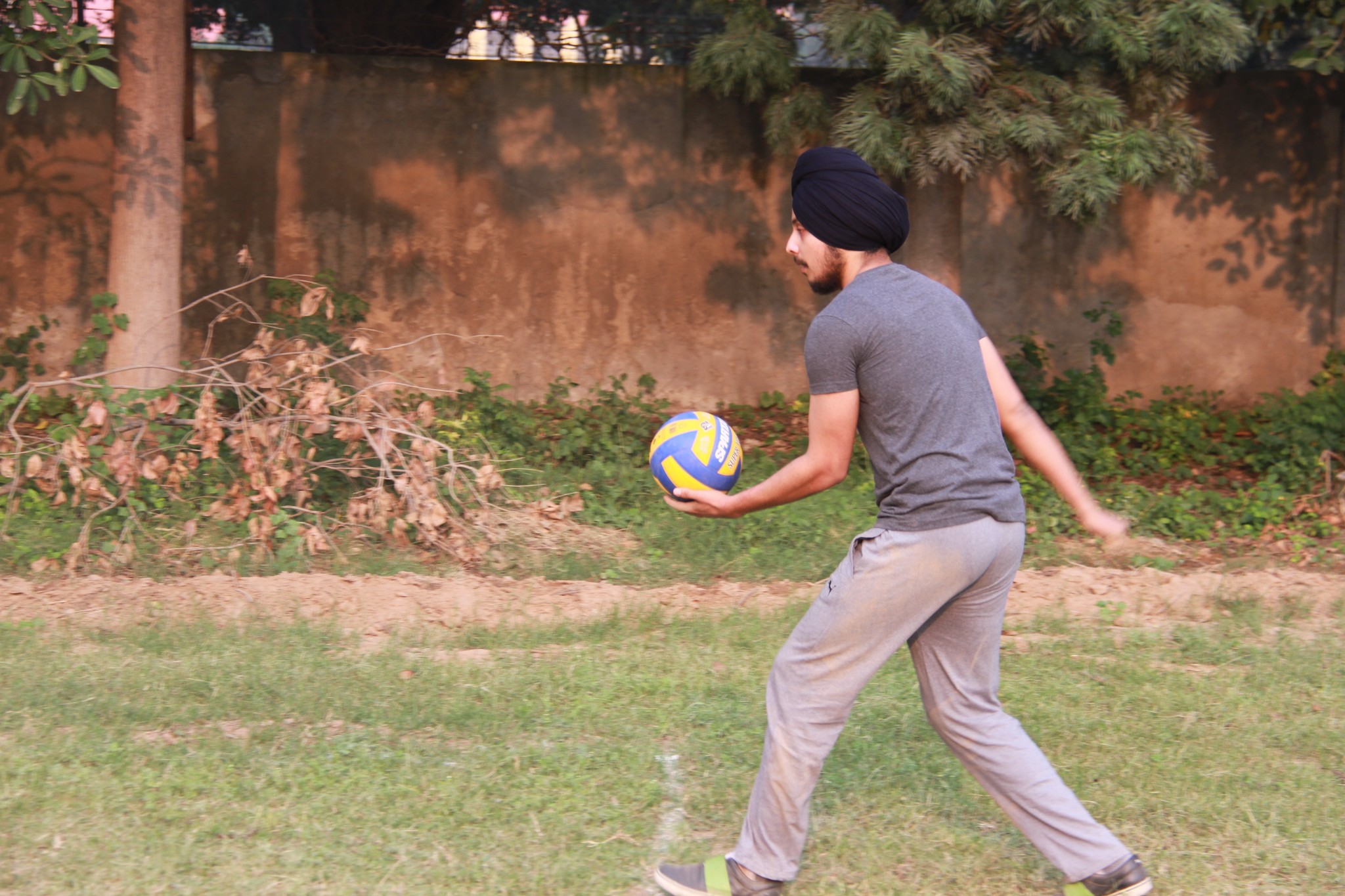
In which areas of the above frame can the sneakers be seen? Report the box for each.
[653,856,784,896]
[1056,855,1153,896]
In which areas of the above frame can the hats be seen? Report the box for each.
[790,146,909,254]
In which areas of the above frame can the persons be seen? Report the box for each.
[651,144,1153,896]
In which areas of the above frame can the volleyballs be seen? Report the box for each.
[650,412,743,502]
